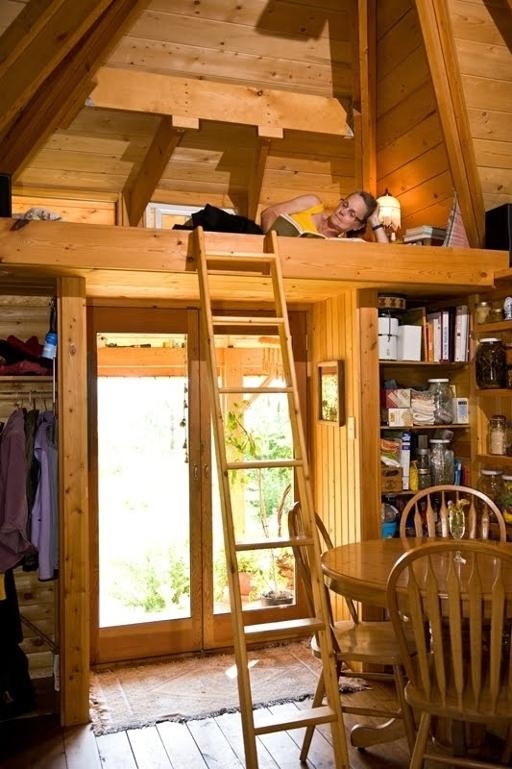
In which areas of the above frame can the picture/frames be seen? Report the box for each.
[316,359,345,426]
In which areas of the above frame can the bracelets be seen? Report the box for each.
[371,223,383,231]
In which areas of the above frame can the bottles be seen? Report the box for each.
[474,302,490,325]
[475,468,512,524]
[485,415,512,456]
[416,439,455,490]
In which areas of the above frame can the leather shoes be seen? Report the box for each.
[0,358,50,376]
[7,335,47,364]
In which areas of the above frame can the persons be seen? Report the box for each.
[183,190,392,244]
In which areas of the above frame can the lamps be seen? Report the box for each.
[376,188,401,242]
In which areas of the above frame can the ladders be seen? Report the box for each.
[192,226,351,769]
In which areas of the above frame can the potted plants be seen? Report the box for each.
[215,399,294,608]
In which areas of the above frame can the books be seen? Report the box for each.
[405,225,446,237]
[266,211,328,240]
[402,231,446,243]
[401,240,446,246]
[402,302,473,365]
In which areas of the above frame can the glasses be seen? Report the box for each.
[338,198,362,223]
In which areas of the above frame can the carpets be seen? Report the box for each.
[89,637,373,737]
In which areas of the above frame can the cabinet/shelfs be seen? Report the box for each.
[306,287,512,686]
[0,276,89,728]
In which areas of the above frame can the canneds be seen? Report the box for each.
[489,417,507,454]
[418,469,432,489]
[416,449,429,469]
[475,302,492,325]
[477,469,504,522]
[429,439,454,486]
[502,474,512,524]
[490,308,503,322]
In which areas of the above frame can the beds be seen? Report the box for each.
[1,216,512,302]
